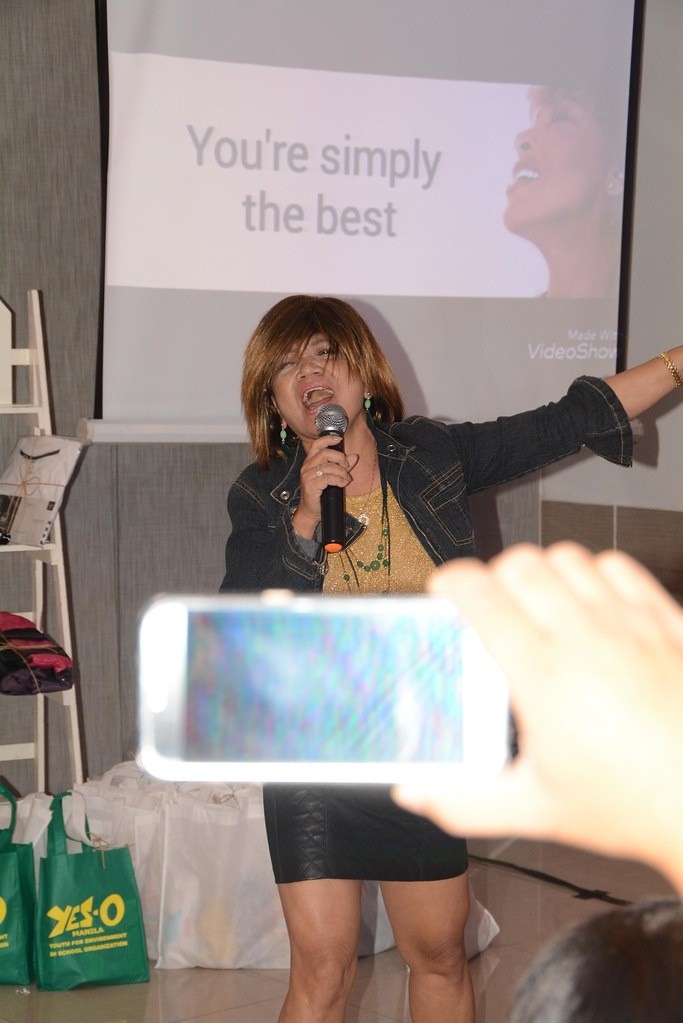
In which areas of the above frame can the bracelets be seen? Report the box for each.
[659,351,683,388]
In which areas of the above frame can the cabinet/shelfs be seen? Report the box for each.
[0,287,86,798]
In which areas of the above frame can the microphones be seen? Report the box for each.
[313,403,349,554]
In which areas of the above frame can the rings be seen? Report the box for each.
[316,464,323,476]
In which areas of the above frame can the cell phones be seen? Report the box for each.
[136,589,509,784]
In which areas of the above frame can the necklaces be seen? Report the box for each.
[339,444,390,595]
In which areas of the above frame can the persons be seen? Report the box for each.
[504,84,625,296]
[389,543,683,900]
[508,899,683,1023]
[219,294,683,1023]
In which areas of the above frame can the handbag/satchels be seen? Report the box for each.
[0,760,499,991]
